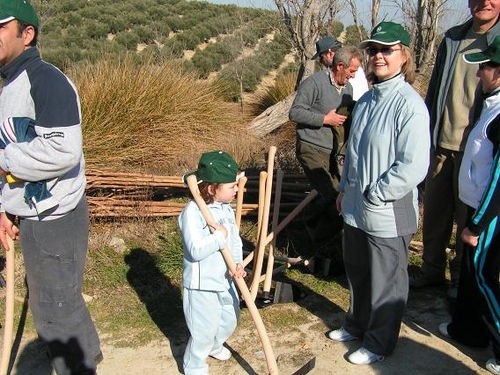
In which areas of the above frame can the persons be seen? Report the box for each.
[177,151,247,375]
[288,0,500,375]
[0,0,104,375]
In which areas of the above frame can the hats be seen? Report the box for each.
[0,0,39,28]
[182,150,245,188]
[310,36,341,60]
[462,35,500,65]
[357,21,411,50]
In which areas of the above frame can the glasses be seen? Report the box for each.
[365,48,402,56]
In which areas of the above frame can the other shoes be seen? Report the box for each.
[211,346,233,361]
[438,322,452,338]
[486,359,500,375]
[448,280,463,304]
[410,271,446,286]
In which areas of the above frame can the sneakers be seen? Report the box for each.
[348,346,384,365]
[329,327,360,341]
